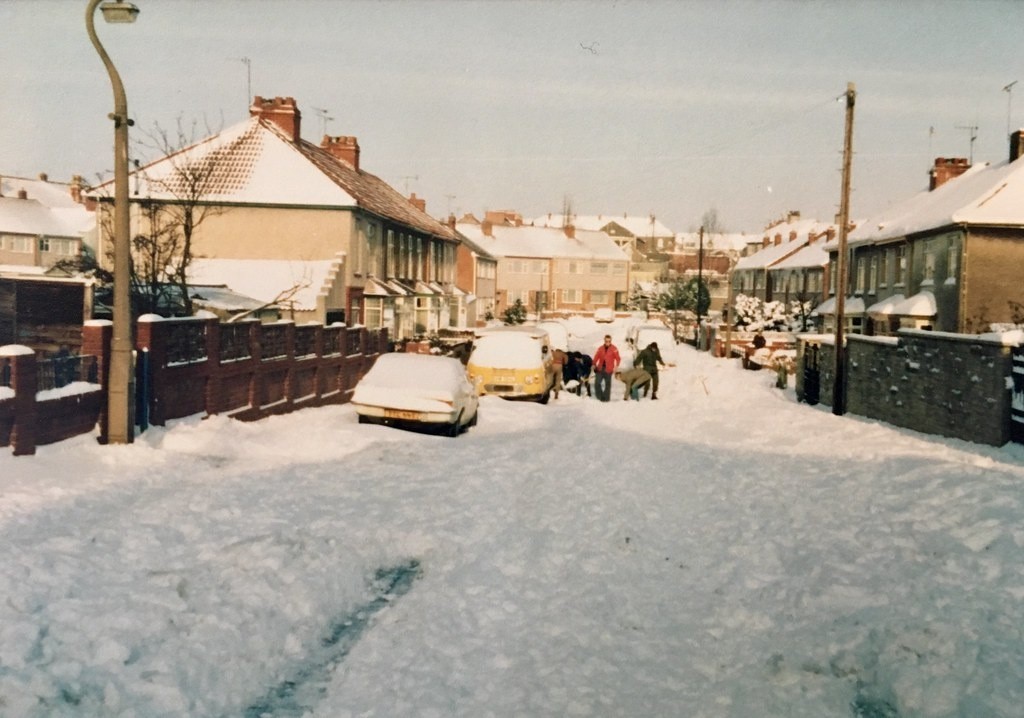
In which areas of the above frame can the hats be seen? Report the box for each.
[650,342,657,349]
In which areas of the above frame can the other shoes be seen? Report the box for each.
[651,394,659,399]
[643,393,647,396]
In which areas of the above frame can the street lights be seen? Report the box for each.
[85,0,142,447]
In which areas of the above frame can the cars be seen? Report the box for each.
[535,305,681,369]
[351,353,480,438]
[466,331,558,408]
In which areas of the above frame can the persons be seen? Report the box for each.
[593,335,620,402]
[752,331,766,349]
[615,368,651,403]
[556,349,593,397]
[633,342,665,399]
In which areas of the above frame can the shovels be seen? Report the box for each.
[566,375,596,388]
